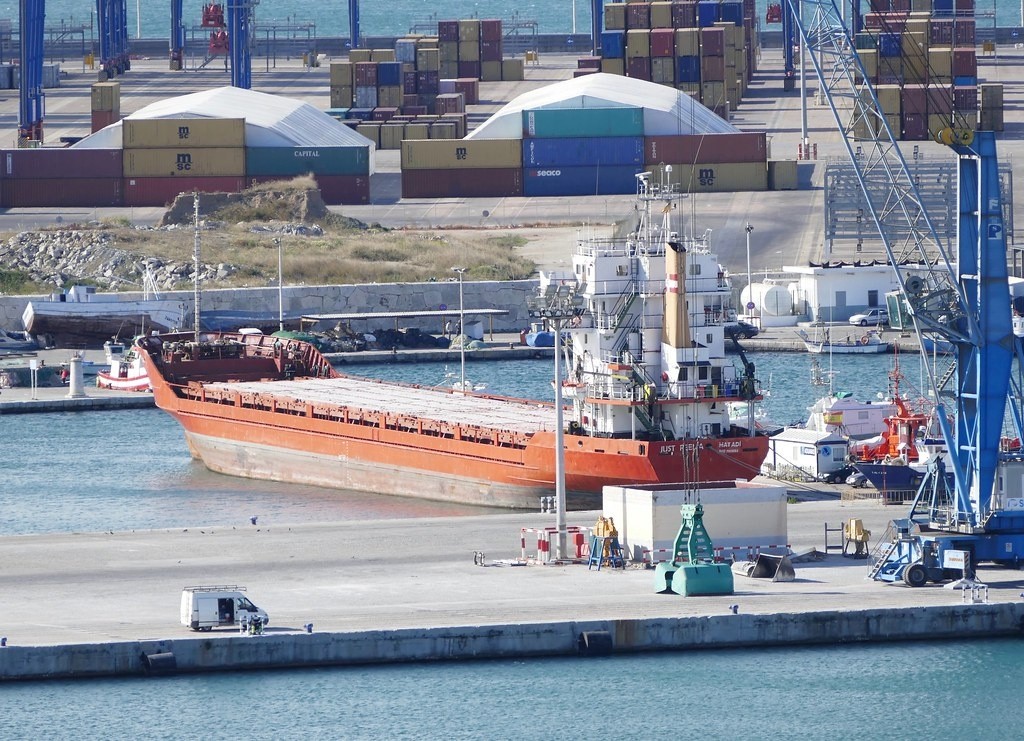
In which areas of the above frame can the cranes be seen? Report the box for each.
[787,0,1024,586]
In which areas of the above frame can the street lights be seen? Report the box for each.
[451,266,470,397]
[526,280,587,560]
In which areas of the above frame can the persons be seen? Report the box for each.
[61,368,68,383]
[446,319,461,341]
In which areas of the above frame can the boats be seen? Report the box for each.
[844,338,1020,505]
[923,336,980,356]
[0,327,40,355]
[186,306,323,335]
[794,319,888,355]
[134,163,769,509]
[21,261,185,341]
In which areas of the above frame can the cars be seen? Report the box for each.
[705,320,758,340]
[818,460,867,488]
[849,307,895,326]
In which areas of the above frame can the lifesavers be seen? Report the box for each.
[860,335,870,345]
